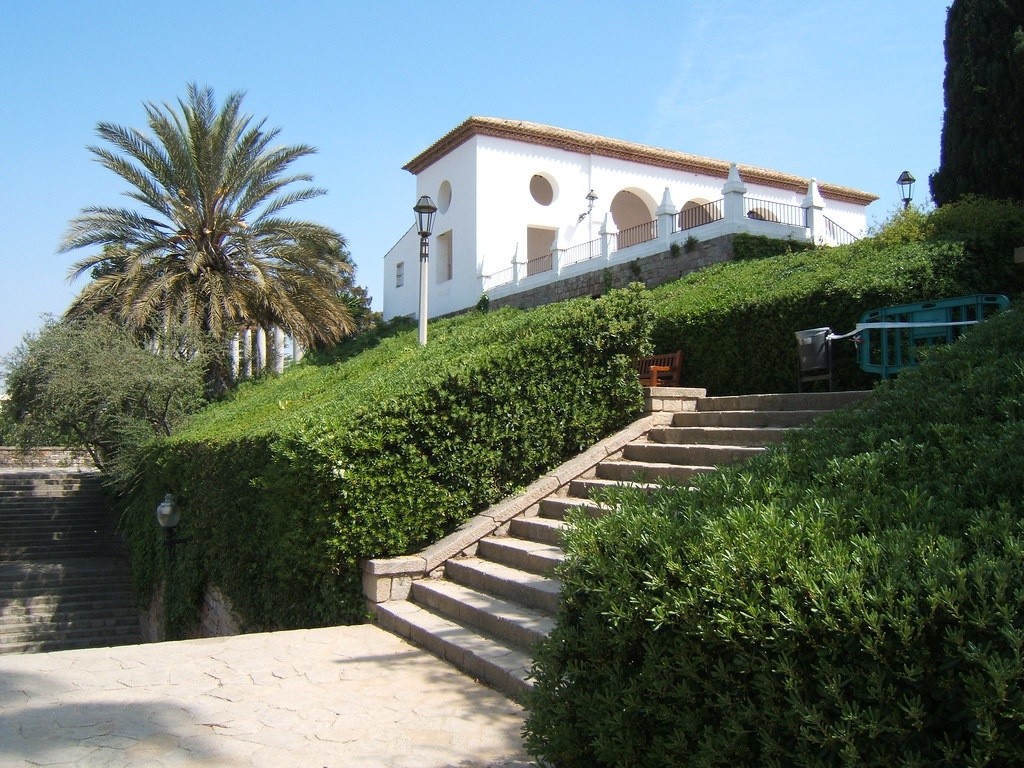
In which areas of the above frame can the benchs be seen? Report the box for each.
[632,350,684,387]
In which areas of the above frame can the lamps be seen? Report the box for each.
[156,494,190,545]
[578,189,598,224]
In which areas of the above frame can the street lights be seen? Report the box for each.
[413,195,438,344]
[896,170,916,210]
[156,489,181,641]
